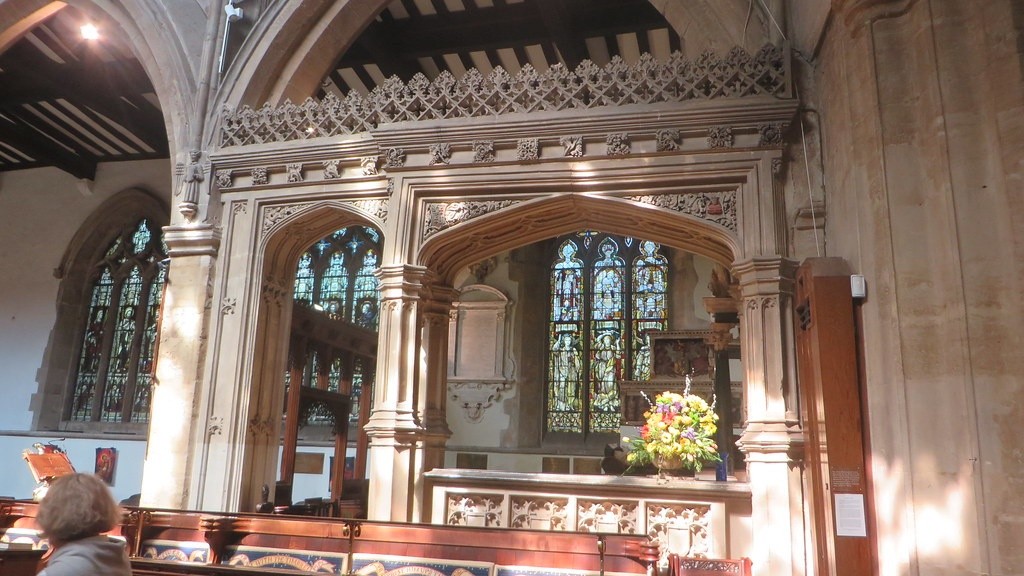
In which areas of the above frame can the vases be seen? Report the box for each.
[651,450,694,470]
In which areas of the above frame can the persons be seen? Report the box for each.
[35,471,137,576]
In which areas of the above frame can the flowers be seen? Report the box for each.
[617,365,720,485]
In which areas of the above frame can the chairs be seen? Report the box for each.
[666,553,753,576]
